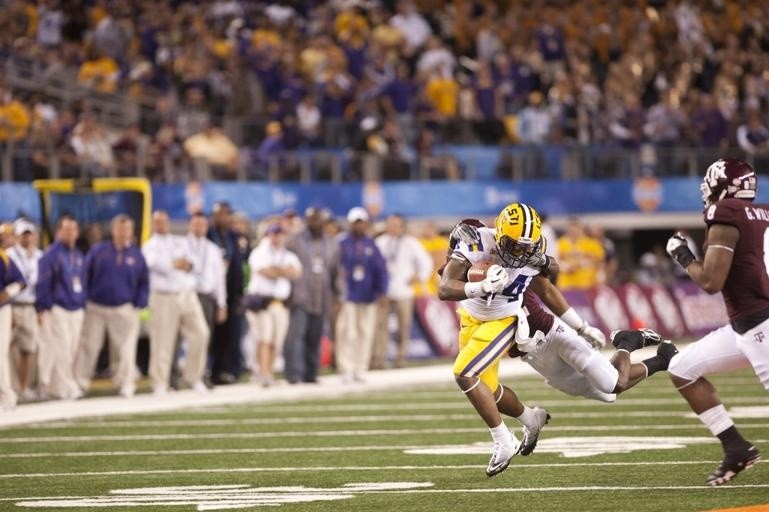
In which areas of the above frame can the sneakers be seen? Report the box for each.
[704,440,760,486]
[520,408,550,457]
[610,328,661,348]
[657,341,678,365]
[486,431,522,477]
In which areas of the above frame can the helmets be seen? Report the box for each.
[701,158,757,213]
[495,203,541,268]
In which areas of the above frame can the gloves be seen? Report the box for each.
[666,233,695,269]
[483,265,509,294]
[449,224,480,244]
[528,249,546,267]
[578,322,606,351]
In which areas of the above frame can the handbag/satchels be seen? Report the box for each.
[241,294,271,312]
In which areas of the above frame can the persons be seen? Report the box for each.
[1,0,769,405]
[439,203,558,478]
[664,154,769,485]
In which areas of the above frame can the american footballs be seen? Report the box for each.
[468,263,504,282]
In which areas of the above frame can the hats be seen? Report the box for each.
[15,216,35,237]
[346,206,369,224]
